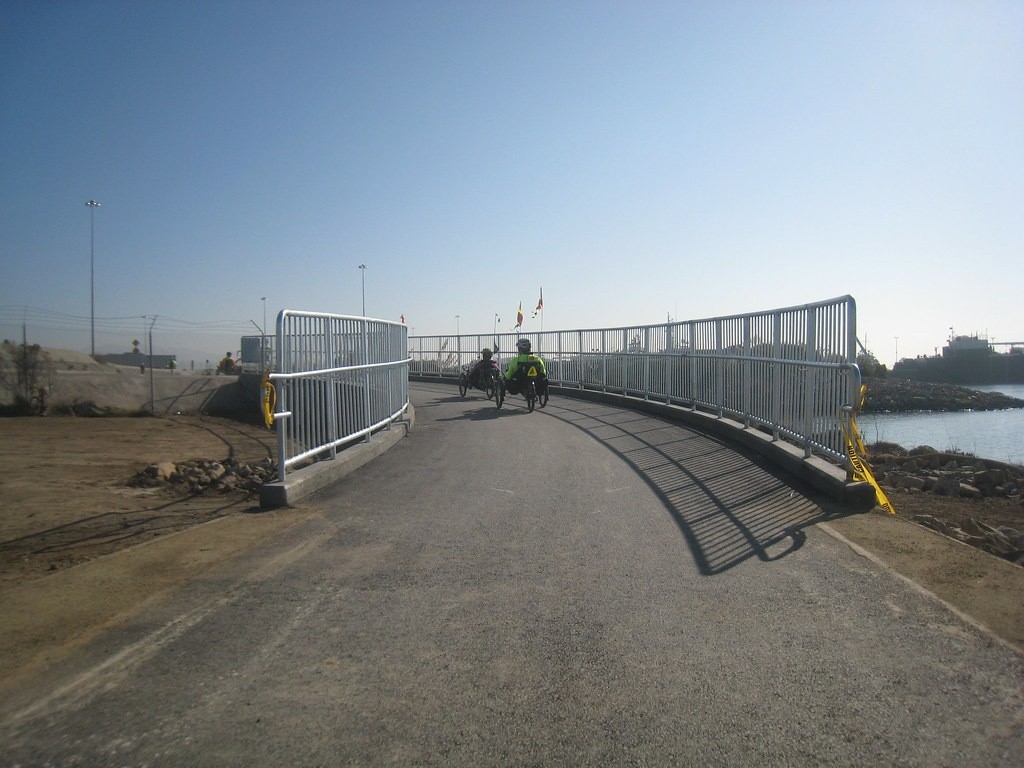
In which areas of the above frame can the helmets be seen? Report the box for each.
[481,348,493,358]
[516,339,531,351]
[227,352,231,356]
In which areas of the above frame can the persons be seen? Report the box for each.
[261,339,271,370]
[503,339,546,395]
[220,352,235,374]
[468,348,501,389]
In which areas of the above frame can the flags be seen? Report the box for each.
[513,304,524,328]
[530,297,543,318]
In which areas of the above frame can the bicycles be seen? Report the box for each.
[495,374,550,412]
[458,366,503,399]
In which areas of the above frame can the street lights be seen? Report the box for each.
[493,312,500,348]
[83,198,102,357]
[260,294,271,343]
[357,261,368,319]
[455,313,463,341]
